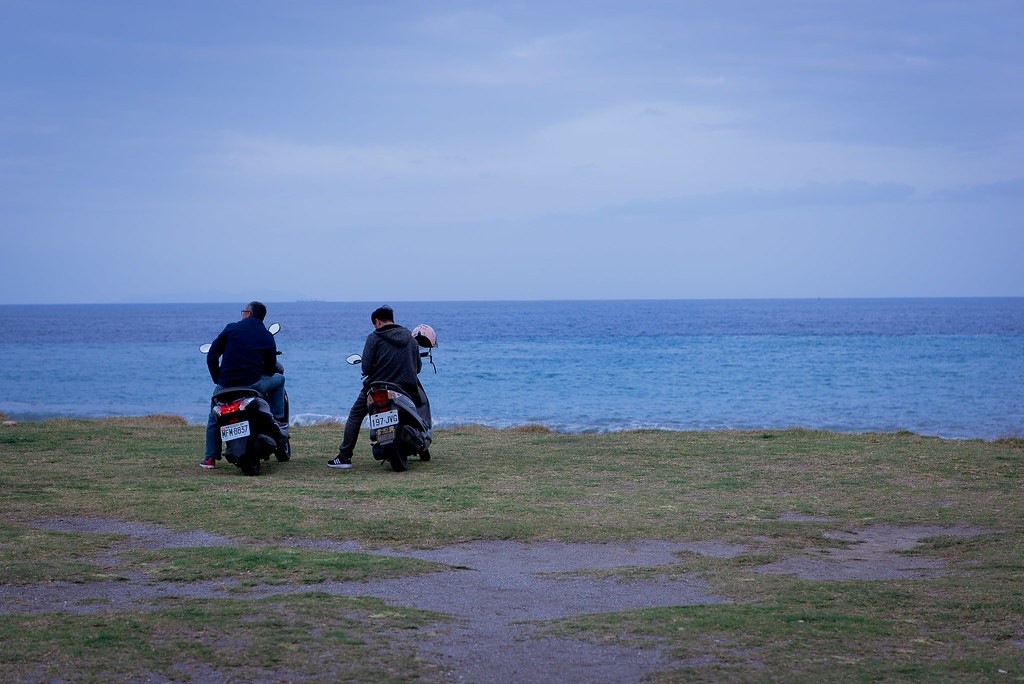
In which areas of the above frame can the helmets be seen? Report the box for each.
[412,324,436,347]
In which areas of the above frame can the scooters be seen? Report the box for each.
[198,323,291,476]
[346,351,431,472]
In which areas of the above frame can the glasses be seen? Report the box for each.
[241,310,252,314]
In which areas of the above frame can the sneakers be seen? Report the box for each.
[276,421,289,429]
[327,454,353,469]
[200,457,216,468]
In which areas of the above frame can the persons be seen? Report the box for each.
[198,301,288,469]
[326,304,428,469]
